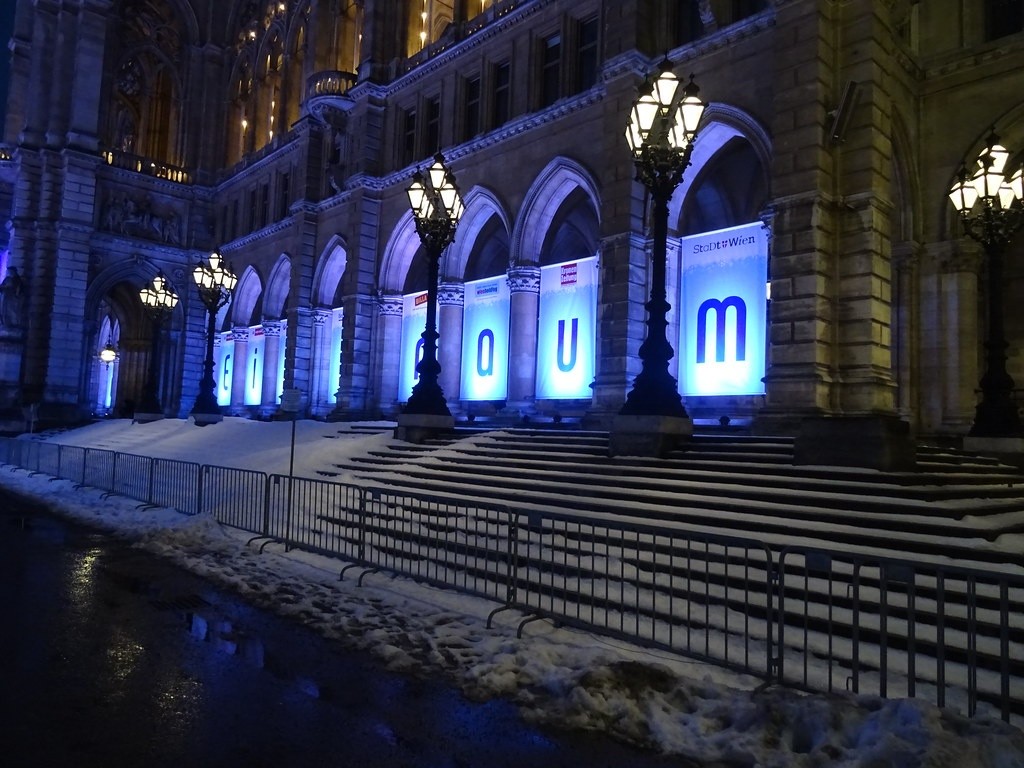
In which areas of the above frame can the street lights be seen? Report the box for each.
[608,49,709,457]
[391,150,466,439]
[194,246,238,421]
[134,271,179,422]
[944,128,1023,452]
